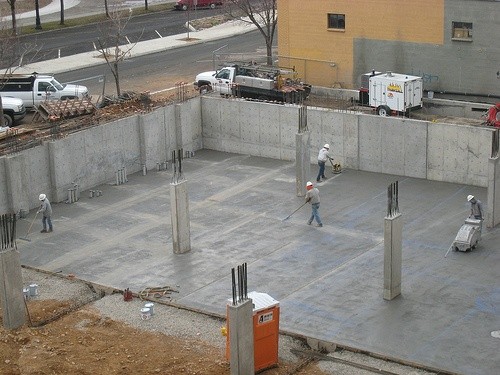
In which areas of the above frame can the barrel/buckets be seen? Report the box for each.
[141,308,150,320]
[428,92,435,99]
[359,89,368,103]
[23,288,30,300]
[29,284,38,295]
[144,303,155,315]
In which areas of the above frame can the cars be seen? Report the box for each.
[1,96,26,128]
[174,0,224,11]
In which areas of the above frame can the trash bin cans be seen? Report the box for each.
[227,291,280,373]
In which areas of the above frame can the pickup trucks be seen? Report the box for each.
[0,75,89,112]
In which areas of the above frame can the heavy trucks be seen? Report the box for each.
[193,63,313,105]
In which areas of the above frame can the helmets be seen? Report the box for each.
[467,194,473,201]
[324,143,330,148]
[306,181,313,186]
[39,193,46,200]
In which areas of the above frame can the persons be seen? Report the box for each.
[37,193,53,233]
[305,181,323,226]
[317,144,334,182]
[467,195,485,221]
